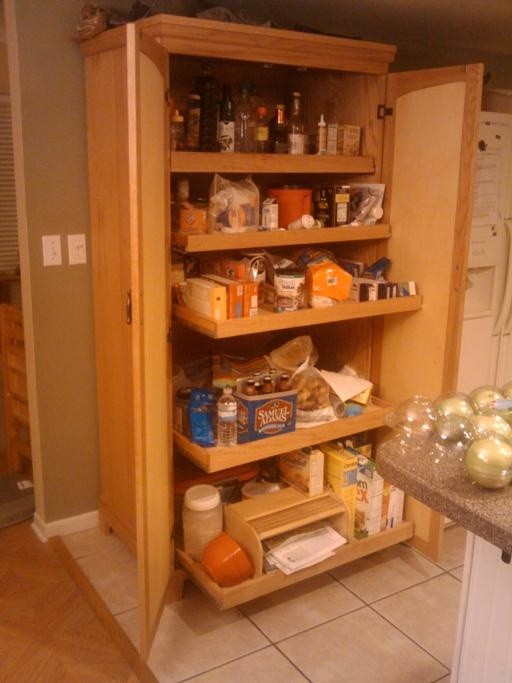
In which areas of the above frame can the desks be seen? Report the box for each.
[372,384,509,683]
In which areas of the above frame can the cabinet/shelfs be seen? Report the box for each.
[80,16,488,658]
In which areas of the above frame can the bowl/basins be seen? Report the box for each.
[203,533,254,586]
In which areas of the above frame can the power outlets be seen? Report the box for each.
[67,234,88,265]
[43,234,62,266]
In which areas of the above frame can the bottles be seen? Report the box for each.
[253,372,261,395]
[182,484,223,561]
[269,368,277,392]
[270,104,290,153]
[243,380,256,395]
[185,62,220,151]
[257,106,269,153]
[171,110,185,150]
[217,387,238,447]
[173,387,192,435]
[315,113,327,153]
[235,86,256,152]
[219,82,235,152]
[287,92,308,154]
[261,376,272,394]
[279,374,288,391]
